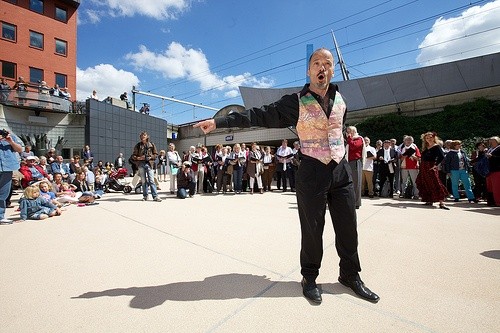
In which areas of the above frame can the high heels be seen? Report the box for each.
[454,198,459,202]
[468,198,480,204]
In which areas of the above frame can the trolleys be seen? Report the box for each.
[102,166,133,193]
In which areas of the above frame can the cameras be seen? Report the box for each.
[185,165,190,168]
[145,155,150,161]
[0,130,9,137]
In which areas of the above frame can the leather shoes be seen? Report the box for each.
[338,275,380,303]
[300,276,322,303]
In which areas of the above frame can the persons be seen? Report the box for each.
[12,76,29,103]
[0,78,11,101]
[193,49,380,305]
[345,126,365,210]
[91,90,99,100]
[363,132,500,209]
[128,130,301,200]
[0,129,126,225]
[120,92,132,109]
[39,80,71,100]
[139,102,150,116]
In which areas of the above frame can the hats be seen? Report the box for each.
[26,155,36,161]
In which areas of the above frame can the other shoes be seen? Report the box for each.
[439,203,451,210]
[369,193,418,200]
[0,219,13,225]
[422,202,434,206]
[142,197,148,201]
[205,187,296,195]
[153,197,164,202]
[187,191,194,198]
